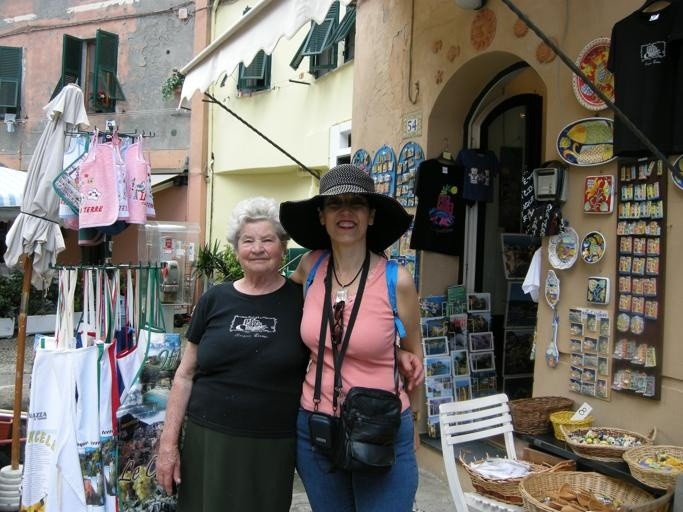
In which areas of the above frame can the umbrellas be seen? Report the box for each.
[1,76,91,469]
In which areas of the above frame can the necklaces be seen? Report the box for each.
[332,258,367,305]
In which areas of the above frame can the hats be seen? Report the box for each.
[279,163,414,251]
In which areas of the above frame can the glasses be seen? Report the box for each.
[332,300,345,344]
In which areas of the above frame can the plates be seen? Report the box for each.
[556,117,619,167]
[548,227,579,270]
[580,231,606,264]
[573,38,616,111]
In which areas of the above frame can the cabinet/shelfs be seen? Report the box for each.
[492,233,543,400]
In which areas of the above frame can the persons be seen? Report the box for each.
[152,195,426,511]
[277,162,426,511]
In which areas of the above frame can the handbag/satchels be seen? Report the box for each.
[338,387,402,471]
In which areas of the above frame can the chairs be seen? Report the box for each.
[439,393,525,512]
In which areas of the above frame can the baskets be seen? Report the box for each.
[465,455,549,506]
[549,410,592,441]
[518,470,656,512]
[622,444,683,490]
[559,423,656,463]
[509,395,573,435]
